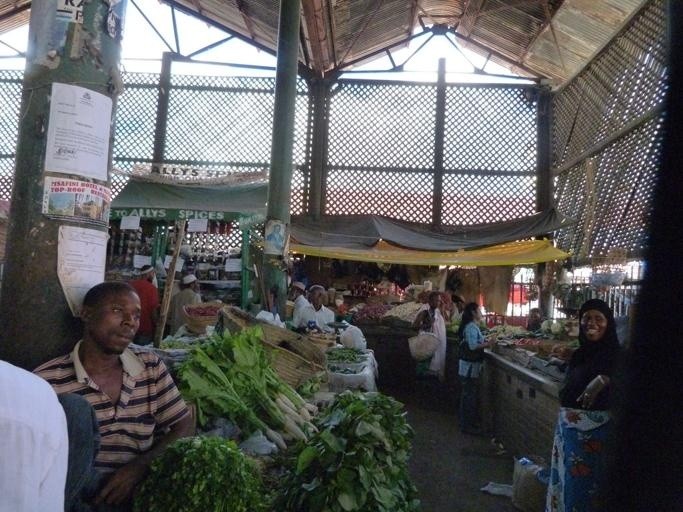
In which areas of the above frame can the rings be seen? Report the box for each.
[584,392,589,397]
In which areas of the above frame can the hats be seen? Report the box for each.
[183,274,197,285]
[292,281,305,292]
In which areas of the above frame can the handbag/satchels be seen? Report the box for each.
[455,326,483,362]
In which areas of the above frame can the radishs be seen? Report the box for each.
[265,392,320,449]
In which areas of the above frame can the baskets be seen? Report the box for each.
[221,306,327,388]
[182,302,224,334]
[511,455,549,512]
[407,324,440,362]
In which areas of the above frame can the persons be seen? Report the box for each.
[526,308,544,334]
[288,281,316,330]
[413,291,447,383]
[168,274,202,338]
[124,265,161,346]
[0,360,70,512]
[456,302,507,456]
[180,245,196,276]
[306,285,334,337]
[32,280,196,511]
[544,298,622,512]
[450,295,465,321]
[266,224,284,253]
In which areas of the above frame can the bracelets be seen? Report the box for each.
[598,373,606,386]
